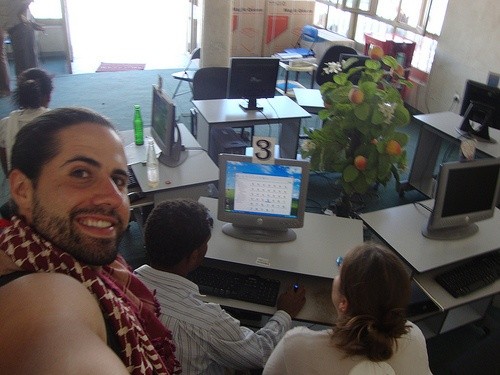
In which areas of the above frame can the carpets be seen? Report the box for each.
[95,61,146,72]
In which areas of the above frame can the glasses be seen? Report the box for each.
[336,255,344,268]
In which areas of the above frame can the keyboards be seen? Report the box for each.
[435,255,500,299]
[126,169,139,188]
[186,268,280,307]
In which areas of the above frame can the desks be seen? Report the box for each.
[116,87,500,341]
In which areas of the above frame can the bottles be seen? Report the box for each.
[146,141,159,186]
[134,105,144,145]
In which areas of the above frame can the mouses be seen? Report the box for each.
[293,283,300,292]
[128,192,146,201]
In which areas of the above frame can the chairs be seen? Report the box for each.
[172,25,358,154]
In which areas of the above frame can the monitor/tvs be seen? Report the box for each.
[215,153,310,242]
[228,56,282,111]
[458,81,500,143]
[422,158,500,240]
[148,84,188,167]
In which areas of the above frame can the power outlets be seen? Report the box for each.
[453,91,461,103]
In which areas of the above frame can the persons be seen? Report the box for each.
[0,108,183,374]
[261,244,437,375]
[0,67,55,181]
[133,197,309,375]
